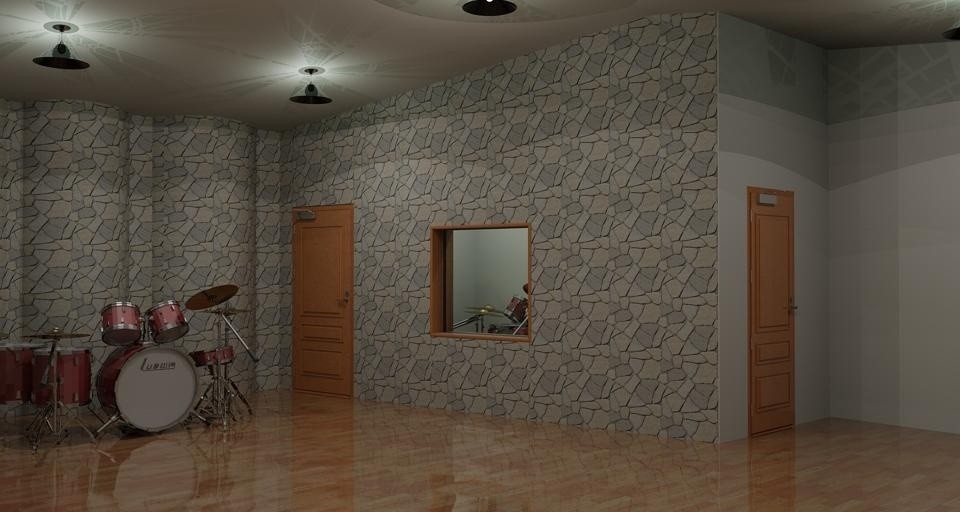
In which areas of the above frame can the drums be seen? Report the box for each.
[96,343,201,433]
[505,296,528,324]
[204,346,235,367]
[511,326,528,336]
[145,299,190,343]
[0,341,46,405]
[101,301,142,345]
[33,347,92,408]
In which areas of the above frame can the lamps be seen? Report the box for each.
[32,23,90,70]
[462,0,517,16]
[288,70,333,104]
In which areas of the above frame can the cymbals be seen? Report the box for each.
[466,303,505,314]
[184,284,238,310]
[469,312,505,319]
[21,331,91,339]
[197,308,251,316]
